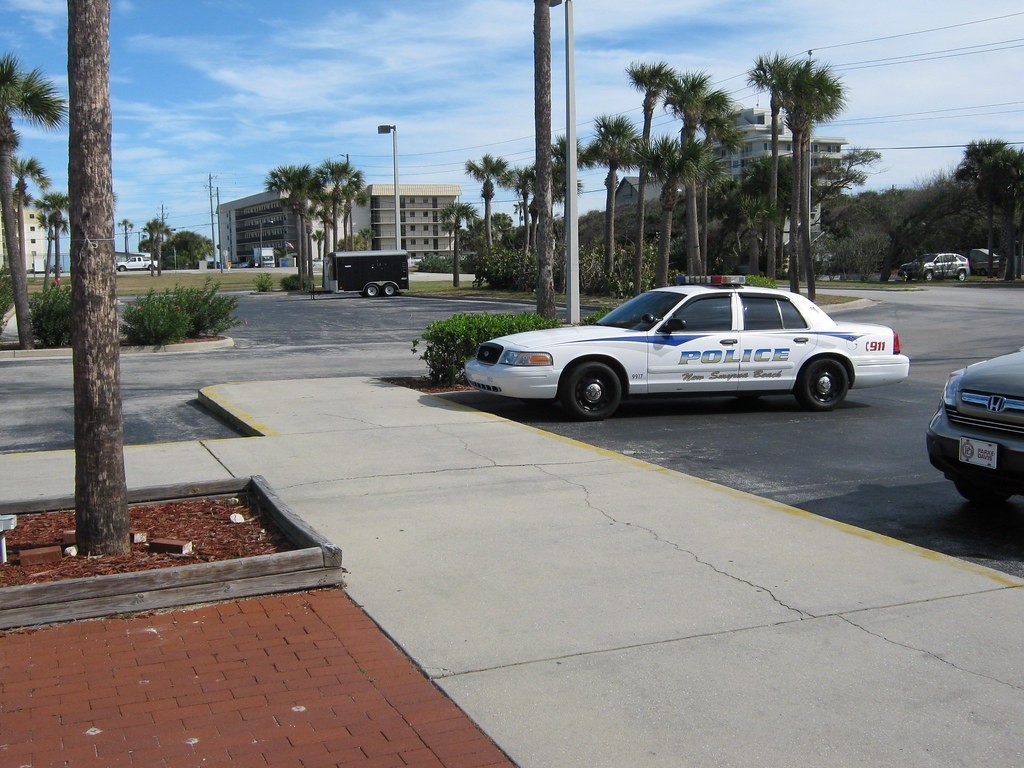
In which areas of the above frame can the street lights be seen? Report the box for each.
[378,125,401,250]
[260,221,274,268]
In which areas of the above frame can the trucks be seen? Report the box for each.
[253,248,275,268]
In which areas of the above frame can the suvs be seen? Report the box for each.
[898,253,970,281]
[207,261,220,269]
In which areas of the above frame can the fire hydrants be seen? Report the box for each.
[902,275,907,282]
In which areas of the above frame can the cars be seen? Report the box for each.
[926,344,1023,505]
[465,275,910,421]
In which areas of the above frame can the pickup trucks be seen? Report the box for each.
[116,257,158,272]
[970,261,1000,276]
